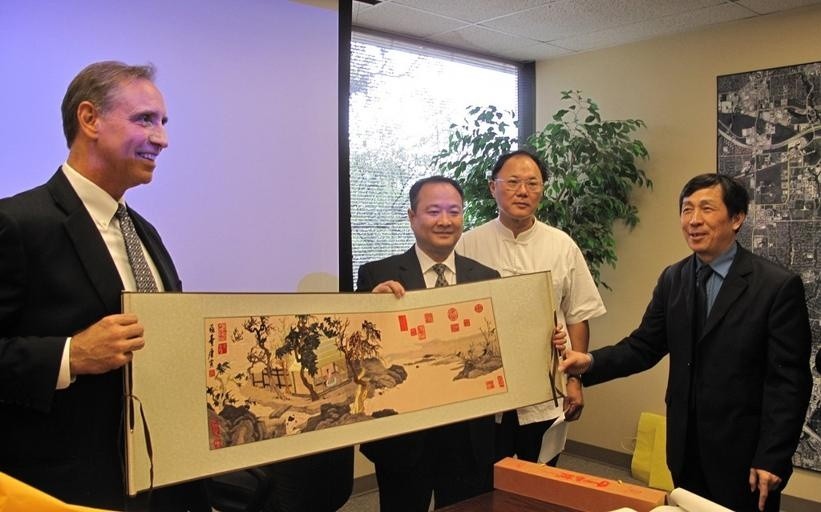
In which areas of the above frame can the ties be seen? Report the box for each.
[695,264,716,341]
[114,201,160,292]
[433,263,450,288]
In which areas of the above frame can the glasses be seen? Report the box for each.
[495,176,545,193]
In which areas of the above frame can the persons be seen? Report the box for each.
[454,150,607,467]
[354,176,567,512]
[558,173,813,512]
[0,61,212,512]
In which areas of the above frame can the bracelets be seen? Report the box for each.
[567,374,581,380]
